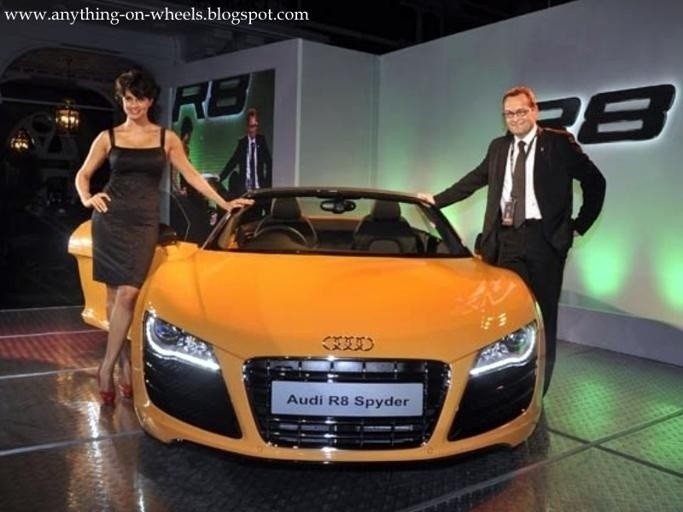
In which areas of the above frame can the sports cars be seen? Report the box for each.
[68,185,547,467]
[183,174,231,223]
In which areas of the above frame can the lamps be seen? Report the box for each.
[9,126,34,155]
[52,96,82,139]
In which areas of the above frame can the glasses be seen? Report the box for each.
[503,110,529,117]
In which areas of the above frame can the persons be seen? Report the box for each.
[418,86,606,398]
[212,108,272,196]
[172,116,193,197]
[75,69,255,405]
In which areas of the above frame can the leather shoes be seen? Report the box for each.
[118,381,132,397]
[96,363,115,404]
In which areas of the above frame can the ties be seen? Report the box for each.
[512,141,526,230]
[250,142,255,189]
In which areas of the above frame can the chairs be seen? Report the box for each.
[350,195,424,256]
[254,196,319,247]
